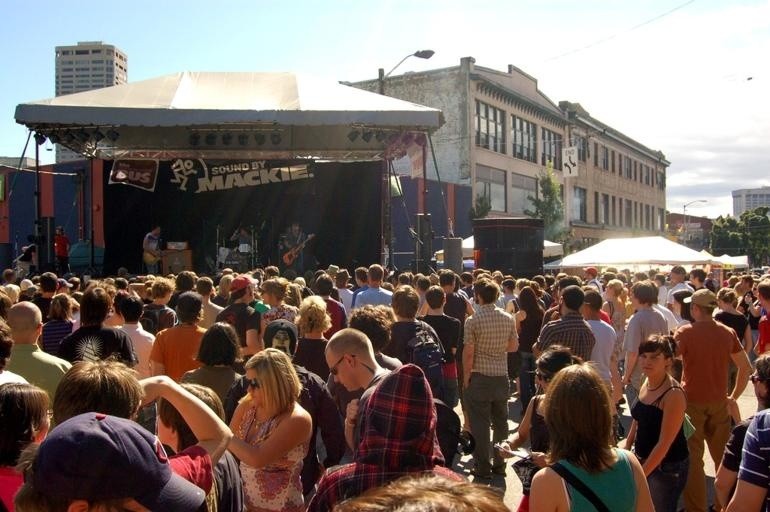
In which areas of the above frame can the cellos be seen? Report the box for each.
[282,233,317,266]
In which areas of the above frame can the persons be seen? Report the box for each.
[0,383,53,512]
[582,287,611,324]
[729,268,770,365]
[2,269,17,287]
[672,290,695,328]
[104,278,114,285]
[623,334,690,512]
[646,280,680,332]
[325,327,404,451]
[114,277,128,290]
[30,272,57,324]
[211,275,235,307]
[690,268,707,288]
[112,296,155,382]
[53,358,234,496]
[68,277,81,292]
[305,363,470,512]
[214,268,234,288]
[532,285,596,364]
[56,277,73,294]
[461,282,520,478]
[264,266,280,281]
[138,276,177,335]
[672,288,753,512]
[6,284,21,304]
[330,471,508,512]
[284,221,312,274]
[0,315,29,388]
[582,290,619,418]
[167,271,196,310]
[528,364,658,512]
[315,274,348,339]
[195,276,225,329]
[513,285,545,414]
[248,299,269,314]
[712,352,770,508]
[149,291,208,455]
[17,234,37,277]
[283,264,632,307]
[32,275,42,288]
[633,272,648,282]
[251,269,264,284]
[602,279,628,410]
[155,383,244,512]
[142,224,163,275]
[70,292,83,334]
[260,277,299,337]
[498,345,583,512]
[706,271,715,292]
[649,269,658,280]
[419,268,475,430]
[54,290,138,370]
[223,319,348,469]
[228,348,314,512]
[232,272,239,278]
[386,285,446,400]
[231,225,253,270]
[667,266,695,323]
[215,276,262,362]
[712,408,770,512]
[0,286,11,321]
[621,282,670,408]
[53,224,70,275]
[118,267,128,278]
[86,280,98,291]
[505,278,531,399]
[19,288,37,302]
[655,274,667,305]
[104,290,129,328]
[180,322,244,403]
[415,285,462,410]
[712,287,753,428]
[20,279,33,290]
[42,293,73,354]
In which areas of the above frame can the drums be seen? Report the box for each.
[219,247,230,257]
[238,244,252,254]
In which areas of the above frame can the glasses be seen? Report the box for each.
[751,374,765,383]
[328,353,354,375]
[41,410,55,419]
[536,372,557,384]
[241,374,261,392]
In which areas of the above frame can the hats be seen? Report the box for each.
[263,320,298,346]
[335,269,352,279]
[683,289,719,306]
[229,275,251,292]
[324,265,340,274]
[583,266,599,276]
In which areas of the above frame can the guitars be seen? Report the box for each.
[142,249,177,266]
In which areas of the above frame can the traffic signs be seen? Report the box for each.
[562,148,579,177]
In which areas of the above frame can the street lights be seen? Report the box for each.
[684,199,708,231]
[33,133,46,244]
[378,50,435,94]
